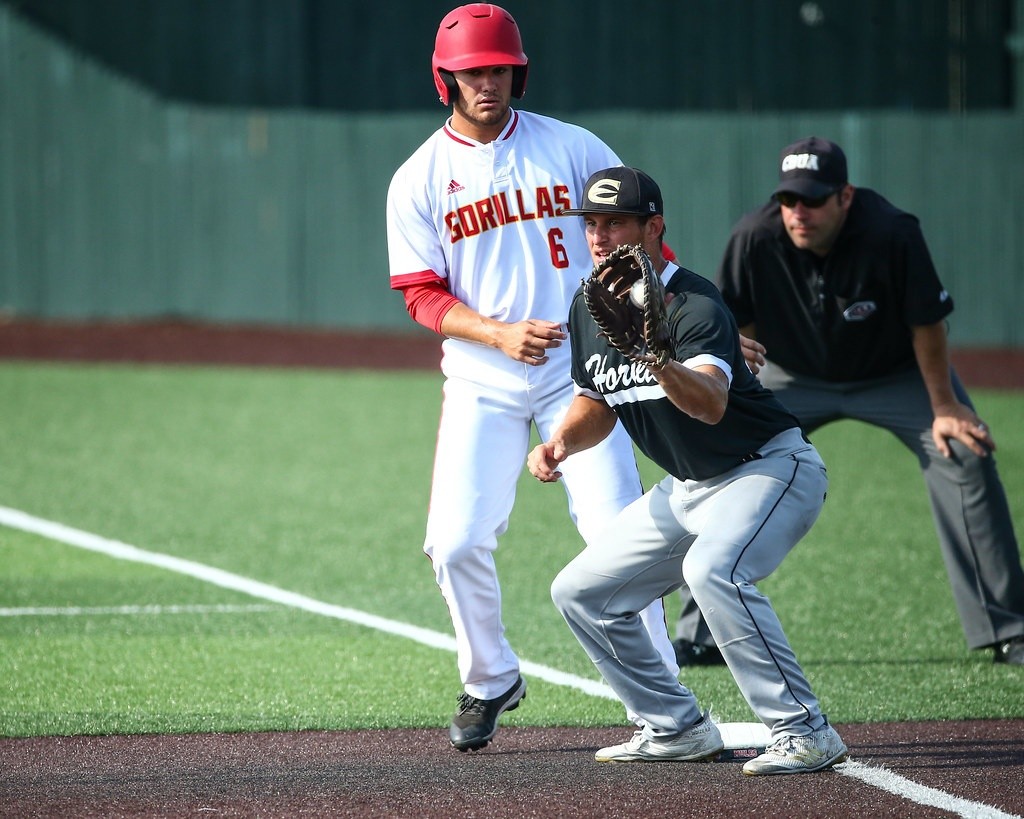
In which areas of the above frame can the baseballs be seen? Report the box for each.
[629,278,644,310]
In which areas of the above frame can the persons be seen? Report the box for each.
[675,135,1024,670]
[528,165,847,775]
[387,3,767,754]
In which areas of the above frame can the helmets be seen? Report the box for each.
[431,4,529,105]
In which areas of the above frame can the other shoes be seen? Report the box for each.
[670,637,728,669]
[994,636,1024,665]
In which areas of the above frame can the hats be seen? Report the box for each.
[772,136,849,203]
[560,166,663,217]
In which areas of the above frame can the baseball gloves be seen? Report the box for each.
[583,245,672,367]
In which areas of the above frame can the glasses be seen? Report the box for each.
[778,192,837,209]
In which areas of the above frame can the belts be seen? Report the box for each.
[741,430,812,464]
[560,322,570,335]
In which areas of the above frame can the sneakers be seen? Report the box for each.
[595,709,725,762]
[742,714,848,775]
[448,673,527,751]
